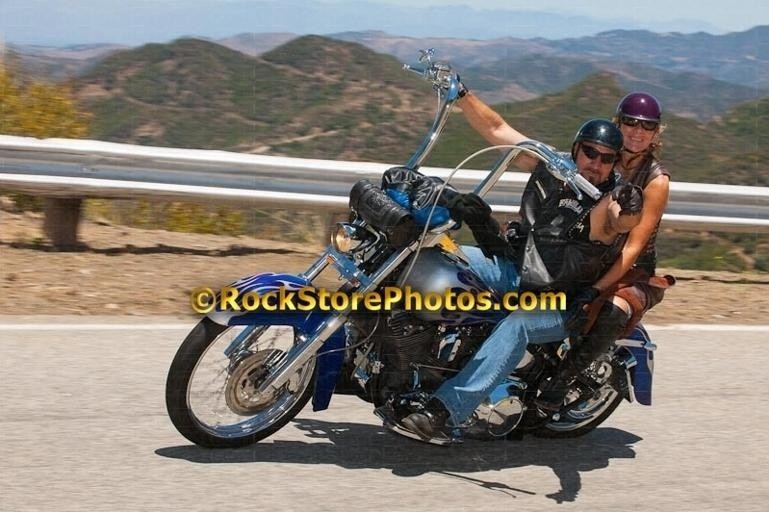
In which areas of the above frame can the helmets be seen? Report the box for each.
[614,90,662,126]
[572,118,623,153]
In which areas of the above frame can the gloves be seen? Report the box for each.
[431,67,468,100]
[564,285,598,329]
[610,184,641,216]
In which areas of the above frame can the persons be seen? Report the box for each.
[532,93,676,414]
[401,62,644,443]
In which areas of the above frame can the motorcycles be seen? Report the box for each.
[165,48,657,450]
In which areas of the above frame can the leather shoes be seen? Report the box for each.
[400,397,451,441]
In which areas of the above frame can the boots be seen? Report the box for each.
[533,298,629,412]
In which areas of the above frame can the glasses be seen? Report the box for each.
[579,141,616,164]
[619,112,657,132]
